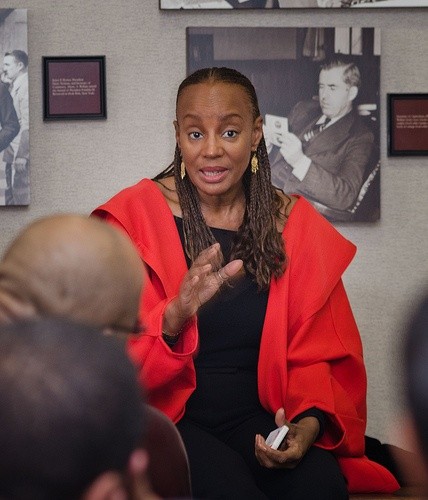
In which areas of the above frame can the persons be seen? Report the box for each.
[86,66,399,500]
[390,285,428,500]
[1,211,148,357]
[0,49,30,206]
[260,62,375,211]
[1,312,154,500]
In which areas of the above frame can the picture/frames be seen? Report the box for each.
[386,92,428,158]
[42,55,108,122]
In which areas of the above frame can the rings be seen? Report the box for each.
[219,268,229,282]
